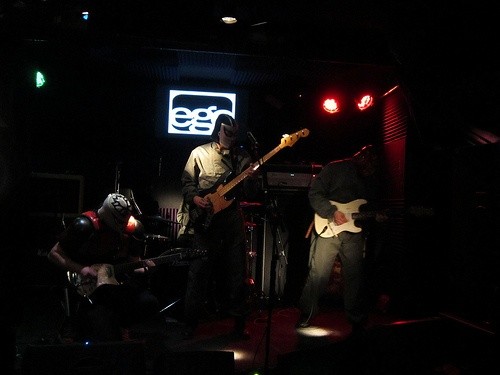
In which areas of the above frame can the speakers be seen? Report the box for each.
[154,352,236,375]
[11,175,84,219]
[275,318,443,375]
[247,210,304,305]
[24,343,147,375]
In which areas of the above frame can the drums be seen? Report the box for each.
[147,235,169,257]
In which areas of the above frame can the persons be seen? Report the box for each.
[178,114,262,339]
[48,193,166,344]
[294,143,388,338]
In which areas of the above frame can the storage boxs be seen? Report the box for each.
[31,172,83,217]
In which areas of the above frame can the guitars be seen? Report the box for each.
[67,245,211,299]
[189,127,310,234]
[313,198,433,239]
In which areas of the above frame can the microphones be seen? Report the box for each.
[54,212,67,228]
[246,131,258,144]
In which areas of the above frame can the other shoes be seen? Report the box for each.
[235,331,249,340]
[182,330,193,339]
[295,307,313,328]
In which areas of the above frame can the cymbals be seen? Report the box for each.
[142,215,180,224]
